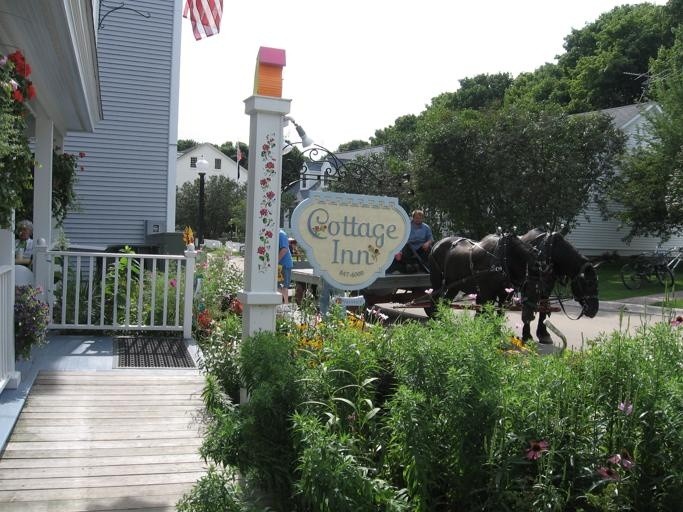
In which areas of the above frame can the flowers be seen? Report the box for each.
[13,284,51,364]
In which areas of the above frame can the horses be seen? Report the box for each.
[425,230,554,347]
[480,228,604,323]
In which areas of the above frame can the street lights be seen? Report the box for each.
[196,152,213,250]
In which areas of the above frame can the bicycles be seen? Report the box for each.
[619,243,682,292]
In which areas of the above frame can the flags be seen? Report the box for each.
[182,0,223,41]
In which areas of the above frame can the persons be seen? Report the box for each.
[12,219,35,271]
[390,208,434,275]
[278,226,293,302]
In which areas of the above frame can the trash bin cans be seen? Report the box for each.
[147,233,185,272]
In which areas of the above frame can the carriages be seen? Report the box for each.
[278,221,600,351]
